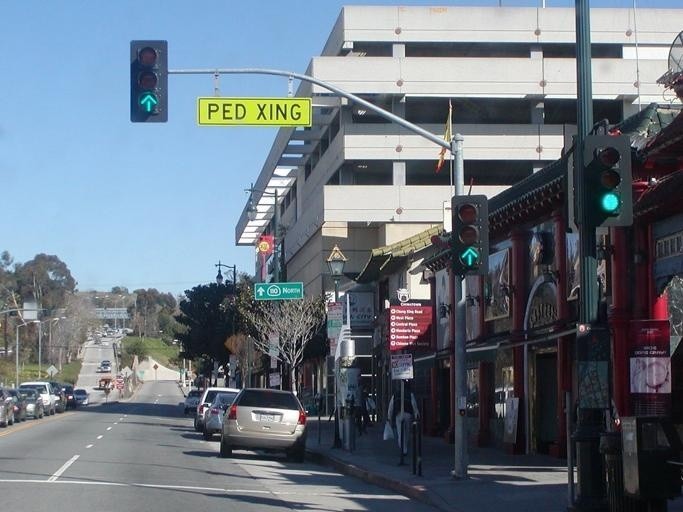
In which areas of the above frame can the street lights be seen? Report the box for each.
[14,320,40,388]
[244,183,280,283]
[48,316,66,367]
[326,243,347,302]
[215,259,236,335]
[37,317,59,380]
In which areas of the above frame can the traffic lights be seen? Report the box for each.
[128,39,169,123]
[582,132,636,229]
[450,193,490,277]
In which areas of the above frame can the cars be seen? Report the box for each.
[99,360,111,372]
[219,387,307,462]
[193,387,242,429]
[98,378,113,390]
[203,391,237,440]
[0,381,88,428]
[183,390,205,415]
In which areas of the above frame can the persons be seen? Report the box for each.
[356,384,369,436]
[388,382,421,454]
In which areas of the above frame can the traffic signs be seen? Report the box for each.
[253,282,304,301]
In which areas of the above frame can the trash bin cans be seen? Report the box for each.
[598,432,667,512]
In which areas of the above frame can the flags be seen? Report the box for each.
[437,104,452,171]
